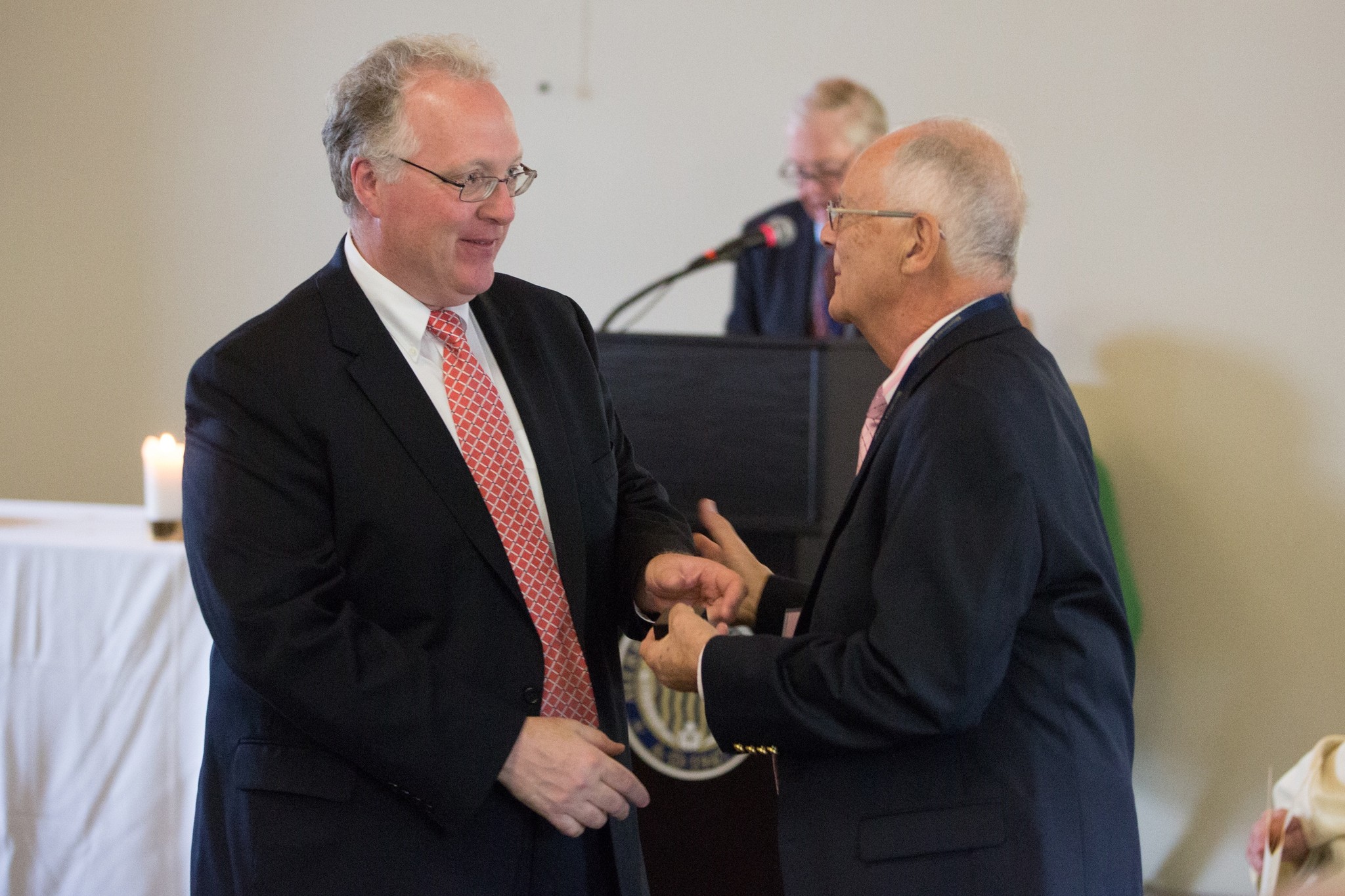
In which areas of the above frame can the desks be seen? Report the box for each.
[2,500,221,895]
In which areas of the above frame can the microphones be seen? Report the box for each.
[597,213,795,333]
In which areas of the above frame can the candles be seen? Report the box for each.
[140,438,180,540]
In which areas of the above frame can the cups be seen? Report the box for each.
[146,452,184,541]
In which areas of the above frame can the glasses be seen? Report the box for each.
[827,202,948,241]
[386,152,539,204]
[774,152,846,184]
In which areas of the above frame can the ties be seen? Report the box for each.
[813,242,848,339]
[426,307,600,725]
[855,383,884,476]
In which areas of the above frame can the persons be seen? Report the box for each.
[639,117,1145,895]
[722,78,891,334]
[179,36,746,895]
